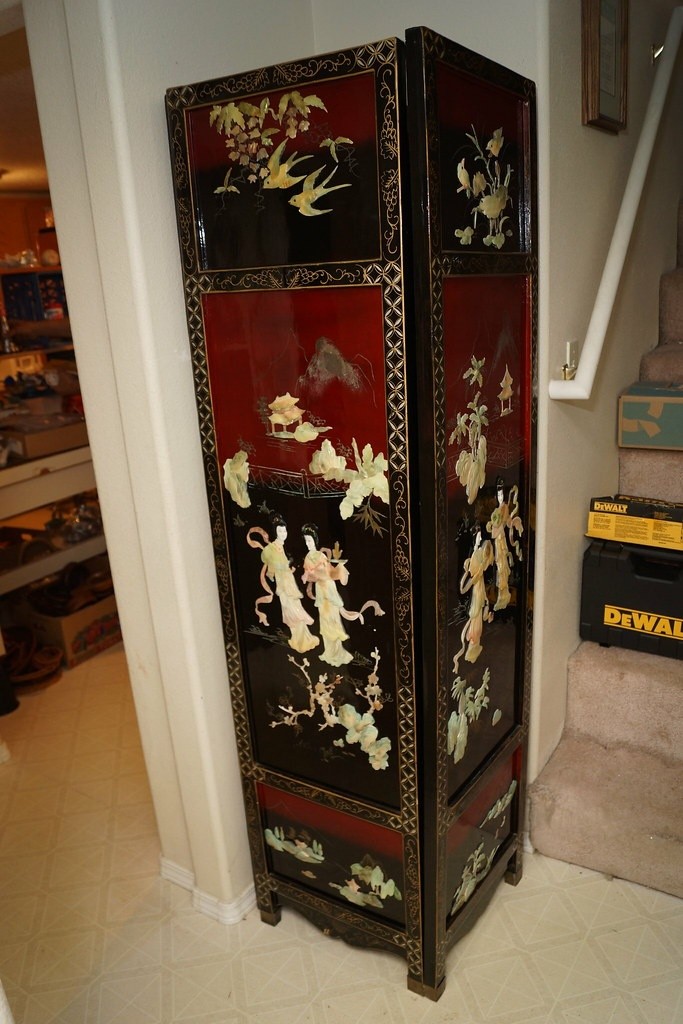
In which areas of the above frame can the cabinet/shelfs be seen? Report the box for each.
[0,449,107,592]
[163,27,537,1000]
[1,265,108,596]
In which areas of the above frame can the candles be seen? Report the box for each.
[566,341,571,367]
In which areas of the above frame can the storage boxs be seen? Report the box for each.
[12,590,121,668]
[580,538,683,661]
[618,382,683,451]
[0,412,88,460]
[585,494,683,552]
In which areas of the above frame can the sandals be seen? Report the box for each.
[0,334,113,684]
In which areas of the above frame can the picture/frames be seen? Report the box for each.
[581,0,630,130]
